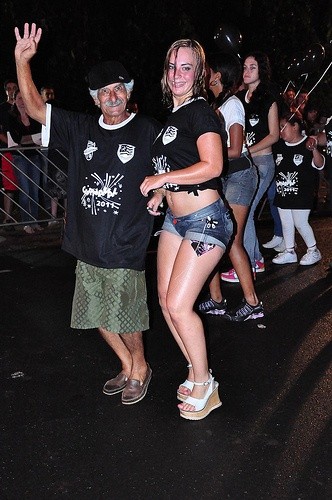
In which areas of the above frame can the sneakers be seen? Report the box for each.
[224,297,264,322]
[271,251,297,264]
[299,249,321,265]
[196,297,227,315]
[255,257,265,273]
[220,266,257,282]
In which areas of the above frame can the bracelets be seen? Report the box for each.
[310,137,318,146]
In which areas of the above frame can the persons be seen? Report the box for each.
[13,23,162,405]
[0,79,332,253]
[140,38,234,421]
[220,54,280,282]
[195,55,264,321]
[273,113,326,266]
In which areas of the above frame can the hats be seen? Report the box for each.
[85,61,132,90]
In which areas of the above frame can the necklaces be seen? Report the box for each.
[178,96,192,107]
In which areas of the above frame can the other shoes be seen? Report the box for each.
[49,220,58,225]
[102,372,127,395]
[262,235,283,248]
[274,239,297,252]
[122,362,153,404]
[23,223,44,233]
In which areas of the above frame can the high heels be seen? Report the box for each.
[177,364,208,402]
[179,374,222,420]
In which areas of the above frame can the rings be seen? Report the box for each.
[309,146,310,148]
